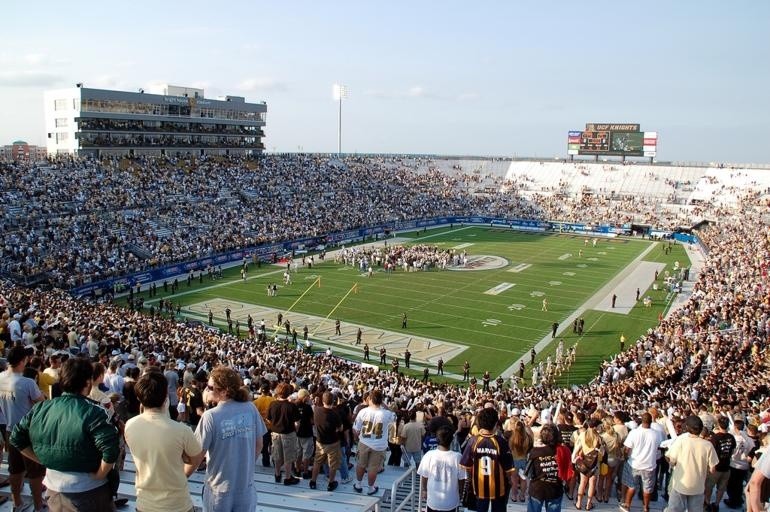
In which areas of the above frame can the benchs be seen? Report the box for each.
[1,162,259,238]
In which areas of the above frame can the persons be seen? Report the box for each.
[2,277,768,511]
[2,111,770,294]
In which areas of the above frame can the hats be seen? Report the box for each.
[13,313,21,320]
[511,408,520,416]
[248,365,256,375]
[264,373,279,382]
[112,350,119,355]
[298,388,310,398]
[243,377,252,386]
[132,350,143,357]
[137,355,147,364]
[565,412,574,423]
[127,354,136,360]
[185,363,196,369]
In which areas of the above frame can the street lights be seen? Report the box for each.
[332,83,351,153]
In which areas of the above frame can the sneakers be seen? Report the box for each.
[347,449,387,474]
[269,468,385,496]
[12,499,49,512]
[704,497,745,512]
[619,503,630,512]
[510,495,526,503]
[196,463,207,473]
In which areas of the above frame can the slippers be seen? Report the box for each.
[564,484,609,511]
[0,477,12,506]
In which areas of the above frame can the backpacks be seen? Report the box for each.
[575,444,598,476]
